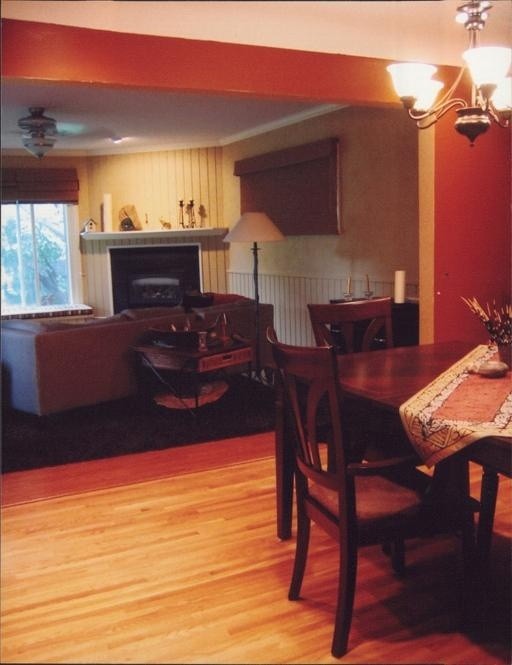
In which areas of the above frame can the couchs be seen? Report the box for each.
[0,291,274,427]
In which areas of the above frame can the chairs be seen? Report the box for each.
[273,339,482,655]
[306,297,390,350]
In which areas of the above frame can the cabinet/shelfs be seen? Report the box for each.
[328,298,418,352]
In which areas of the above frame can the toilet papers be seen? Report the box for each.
[393,270,406,304]
[102,193,112,232]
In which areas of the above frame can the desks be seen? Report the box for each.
[126,344,254,423]
[265,340,512,540]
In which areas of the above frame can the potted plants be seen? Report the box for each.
[460,294,512,371]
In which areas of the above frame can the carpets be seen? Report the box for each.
[1,372,276,474]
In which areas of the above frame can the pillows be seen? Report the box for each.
[1,294,258,333]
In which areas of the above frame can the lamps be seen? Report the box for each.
[15,105,56,159]
[385,0,512,145]
[223,212,287,395]
